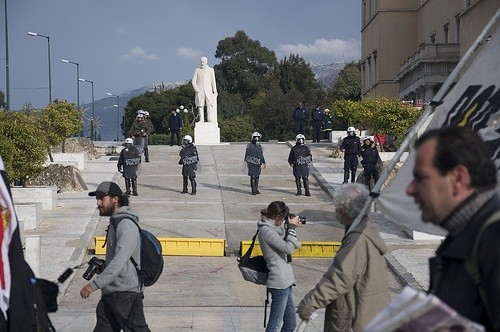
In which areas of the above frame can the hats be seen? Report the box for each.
[315,104,319,108]
[88,181,122,198]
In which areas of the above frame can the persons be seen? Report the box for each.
[288,134,312,196]
[311,105,323,143]
[80,182,151,332]
[406,128,500,332]
[361,136,381,192]
[257,201,301,332]
[168,109,183,146]
[322,109,333,139]
[245,132,266,194]
[297,183,391,332]
[293,102,308,134]
[192,57,218,123]
[340,127,361,184]
[373,131,385,151]
[0,155,35,332]
[179,135,199,194]
[130,110,153,162]
[117,138,142,195]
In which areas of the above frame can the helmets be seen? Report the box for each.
[362,136,370,143]
[183,135,192,143]
[124,138,133,146]
[296,134,305,143]
[135,110,149,121]
[325,108,330,113]
[251,132,261,139]
[347,127,355,134]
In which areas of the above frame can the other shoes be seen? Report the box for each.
[191,192,196,195]
[296,192,302,196]
[256,190,260,194]
[181,192,188,194]
[342,182,348,184]
[313,139,319,142]
[125,191,130,195]
[133,193,138,196]
[305,192,311,196]
[252,192,256,195]
[145,157,149,163]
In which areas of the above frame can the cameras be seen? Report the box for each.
[285,214,306,224]
[83,257,106,281]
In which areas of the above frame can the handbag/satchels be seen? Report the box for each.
[237,255,269,285]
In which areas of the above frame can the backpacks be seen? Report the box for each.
[114,216,164,287]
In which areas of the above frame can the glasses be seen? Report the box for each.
[277,202,286,214]
